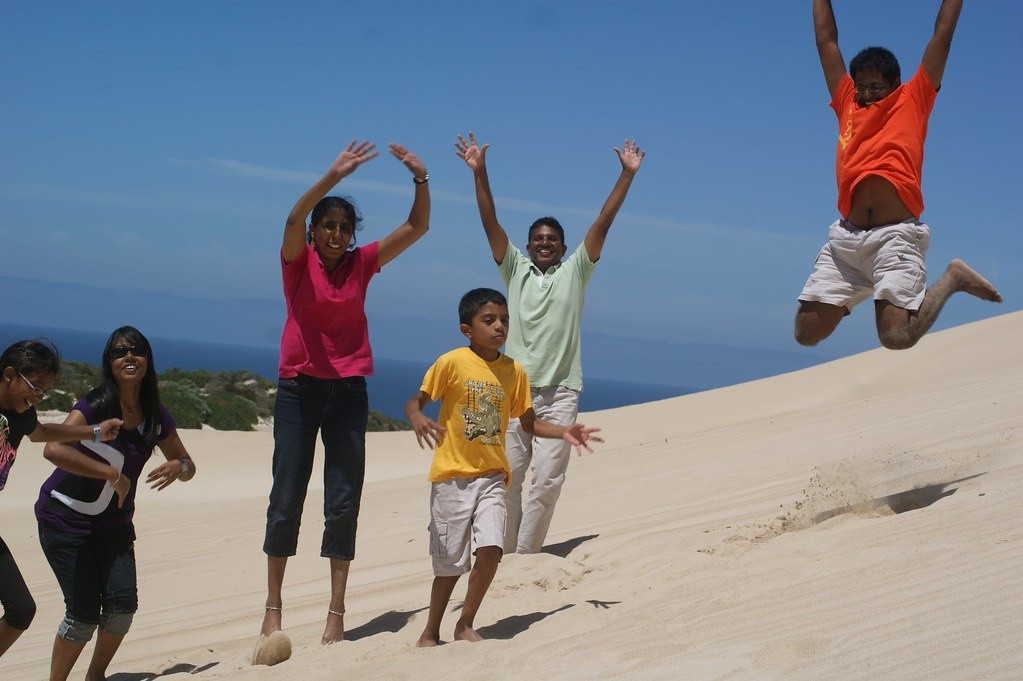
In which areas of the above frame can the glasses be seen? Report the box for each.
[18,371,50,400]
[110,346,147,358]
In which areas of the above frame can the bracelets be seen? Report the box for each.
[412,172,430,184]
[109,469,120,488]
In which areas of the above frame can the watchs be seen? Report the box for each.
[177,457,188,475]
[91,422,101,444]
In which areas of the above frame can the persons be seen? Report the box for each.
[793,0,1004,352]
[404,287,606,650]
[0,339,60,659]
[35,327,198,681]
[450,129,647,555]
[259,139,432,641]
